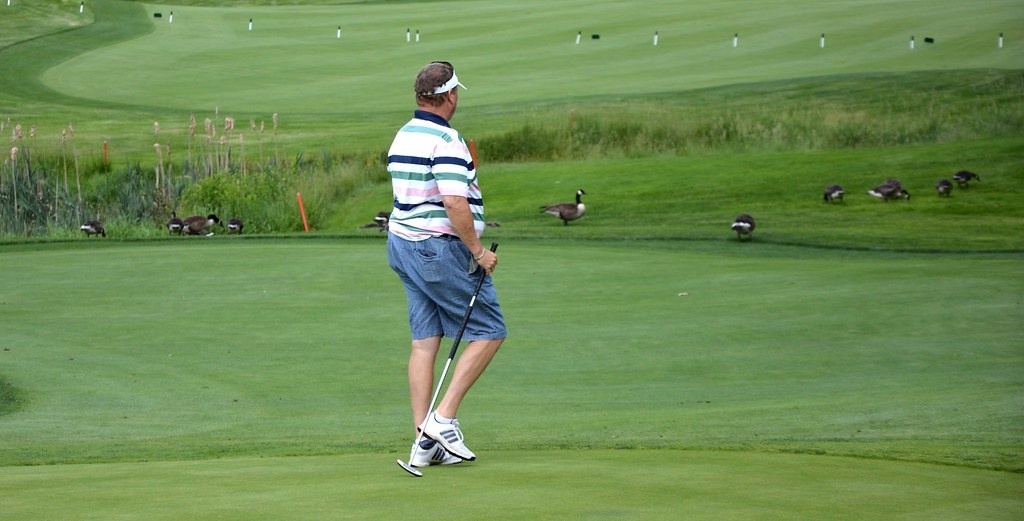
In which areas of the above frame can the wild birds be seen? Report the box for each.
[166,210,244,236]
[866,178,911,203]
[732,213,756,242]
[360,211,392,233]
[951,170,981,189]
[539,189,588,226]
[823,184,845,205]
[80,220,107,239]
[935,178,955,198]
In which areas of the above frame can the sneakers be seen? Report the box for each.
[417,413,476,461]
[410,436,464,466]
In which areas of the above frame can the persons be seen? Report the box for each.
[380,60,507,468]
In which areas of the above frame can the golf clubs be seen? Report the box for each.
[396,240,500,478]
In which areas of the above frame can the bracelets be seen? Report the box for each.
[473,247,486,260]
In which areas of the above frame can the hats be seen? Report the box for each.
[421,61,468,96]
